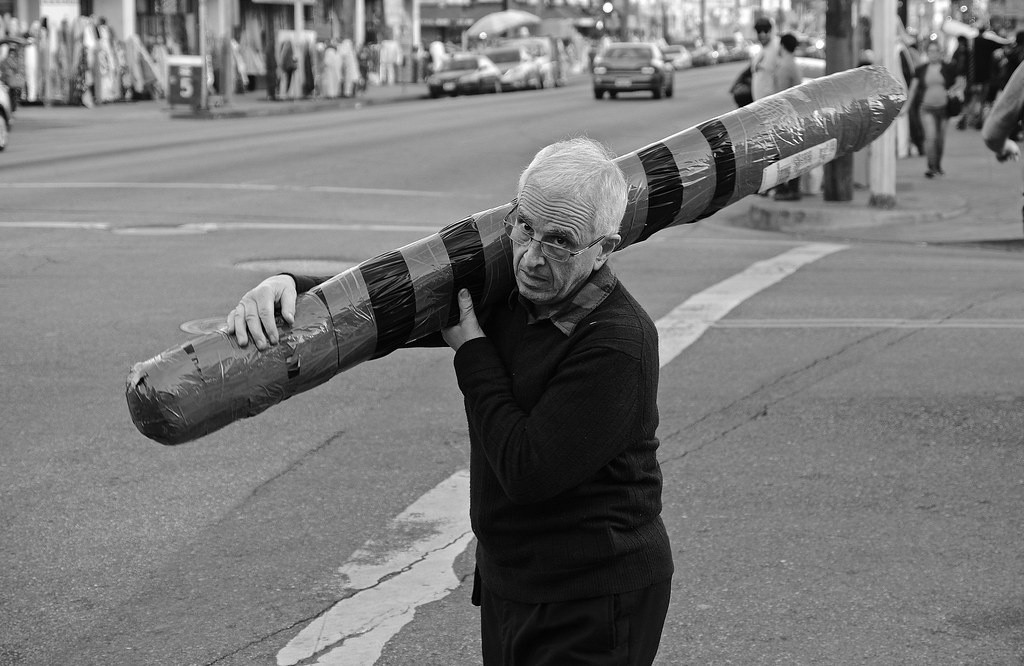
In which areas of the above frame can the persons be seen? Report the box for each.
[0,8,1023,200]
[226,136,675,666]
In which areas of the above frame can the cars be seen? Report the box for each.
[663,36,758,70]
[592,41,675,99]
[425,54,503,97]
[484,35,571,92]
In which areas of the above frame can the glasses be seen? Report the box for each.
[503,202,606,263]
